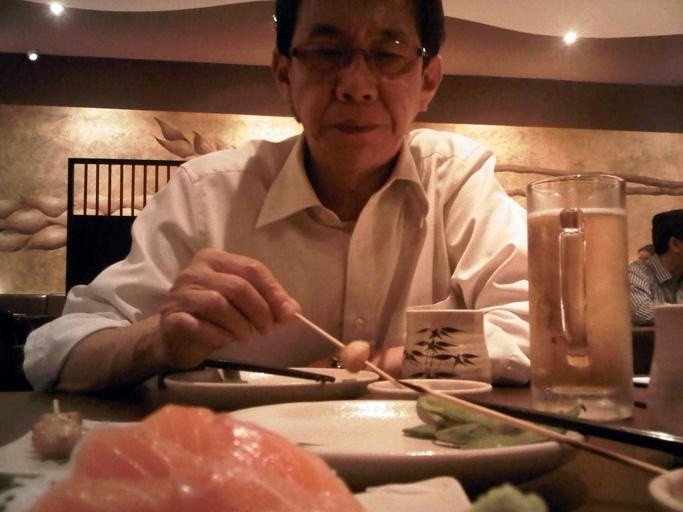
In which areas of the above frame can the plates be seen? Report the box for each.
[158,367,375,409]
[223,397,587,481]
[2,417,150,481]
[366,374,494,408]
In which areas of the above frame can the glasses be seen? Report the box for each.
[291,35,429,76]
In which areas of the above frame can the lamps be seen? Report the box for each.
[24,46,40,65]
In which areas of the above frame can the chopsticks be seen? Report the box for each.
[199,359,334,384]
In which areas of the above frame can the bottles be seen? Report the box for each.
[645,303,682,437]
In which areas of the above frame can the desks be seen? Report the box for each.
[625,323,656,376]
[0,310,60,393]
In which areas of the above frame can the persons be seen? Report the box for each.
[21,0,528,396]
[628,207,682,326]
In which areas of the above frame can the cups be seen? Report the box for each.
[403,307,497,392]
[524,173,631,422]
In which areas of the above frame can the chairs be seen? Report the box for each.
[43,291,69,319]
[0,290,47,316]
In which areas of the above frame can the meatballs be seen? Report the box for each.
[32,411,81,460]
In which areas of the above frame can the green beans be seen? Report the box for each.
[401,395,587,449]
